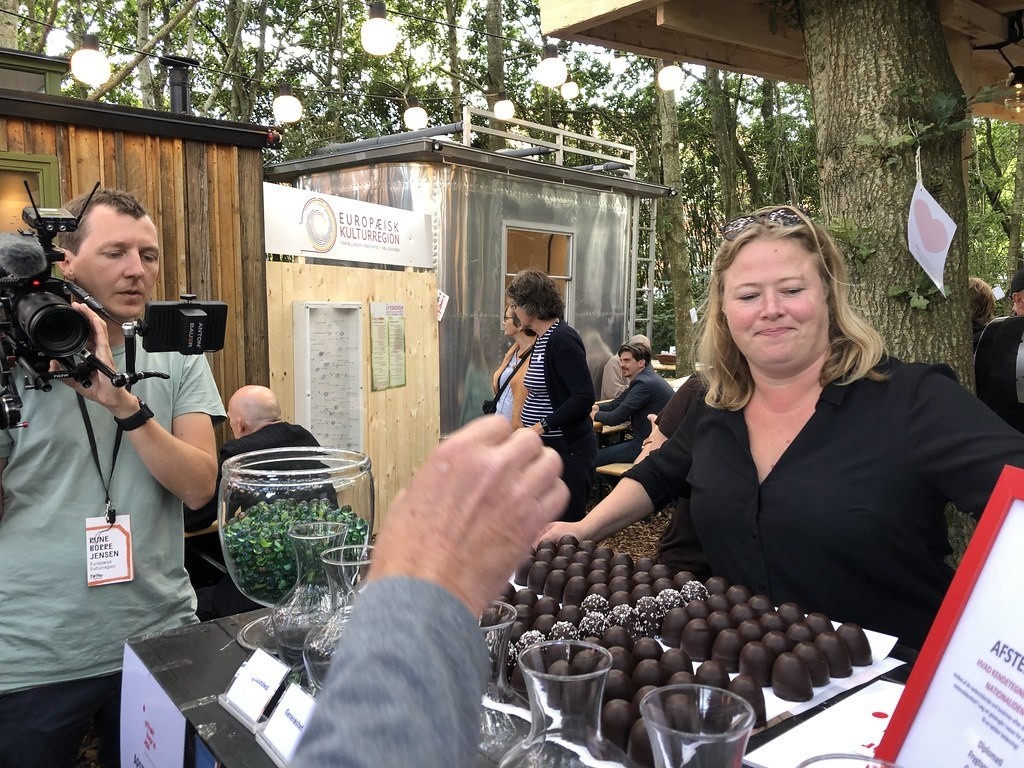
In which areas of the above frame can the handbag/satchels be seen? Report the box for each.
[482,400,496,414]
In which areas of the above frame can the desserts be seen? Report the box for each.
[474,533,872,768]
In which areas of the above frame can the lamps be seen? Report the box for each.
[973,10,1024,113]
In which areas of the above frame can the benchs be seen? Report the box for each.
[594,462,633,505]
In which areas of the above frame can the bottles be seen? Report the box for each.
[497,639,642,768]
[272,522,355,690]
[474,599,533,768]
[302,545,377,700]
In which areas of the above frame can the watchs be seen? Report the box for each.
[114,397,154,431]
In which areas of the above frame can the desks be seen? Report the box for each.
[651,359,714,371]
[591,397,633,435]
[123,605,907,768]
[185,519,220,585]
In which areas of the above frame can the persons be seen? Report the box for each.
[479,267,686,519]
[968,268,1023,434]
[284,412,570,768]
[0,190,227,768]
[491,204,1023,682]
[183,384,339,617]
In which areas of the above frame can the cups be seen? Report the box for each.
[795,753,903,768]
[217,446,375,654]
[639,684,757,768]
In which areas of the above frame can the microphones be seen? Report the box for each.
[0,233,48,278]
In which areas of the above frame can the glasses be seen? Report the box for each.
[718,208,810,242]
[510,303,517,310]
[504,315,514,322]
